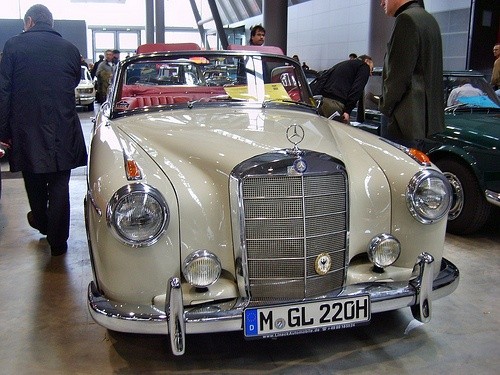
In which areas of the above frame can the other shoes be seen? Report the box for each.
[27,210,47,234]
[50,241,67,255]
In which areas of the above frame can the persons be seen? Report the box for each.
[378,0,445,151]
[0,4,87,256]
[80,49,120,105]
[489,44,500,92]
[236,26,374,124]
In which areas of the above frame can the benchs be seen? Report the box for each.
[121,84,233,109]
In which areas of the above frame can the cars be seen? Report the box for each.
[83,43,469,357]
[350,66,500,237]
[75,65,98,111]
